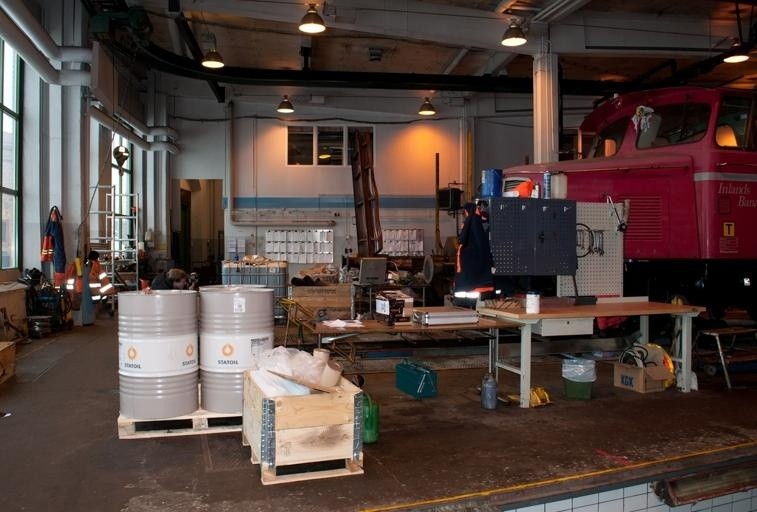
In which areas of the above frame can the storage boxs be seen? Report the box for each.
[241,367,364,486]
[0,341,17,384]
[609,359,676,393]
[394,364,437,398]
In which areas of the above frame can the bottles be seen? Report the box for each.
[533,181,541,199]
[543,169,552,199]
[482,371,498,410]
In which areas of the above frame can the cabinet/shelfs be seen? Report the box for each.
[0,281,32,345]
[290,282,354,322]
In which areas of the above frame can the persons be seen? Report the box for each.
[152,269,188,290]
[86,251,116,295]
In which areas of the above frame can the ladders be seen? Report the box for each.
[348,127,383,257]
[692,326,757,388]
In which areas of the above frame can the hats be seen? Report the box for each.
[165,269,189,279]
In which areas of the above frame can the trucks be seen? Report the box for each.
[490,82,757,347]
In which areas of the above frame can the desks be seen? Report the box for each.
[313,301,527,405]
[478,302,706,409]
[354,281,431,320]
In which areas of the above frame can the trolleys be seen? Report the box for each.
[79,183,142,317]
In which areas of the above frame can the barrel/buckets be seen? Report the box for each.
[118,290,198,421]
[363,392,379,444]
[200,284,276,414]
[480,374,498,409]
[481,169,502,197]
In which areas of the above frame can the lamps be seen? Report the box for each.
[723,37,749,63]
[418,96,437,116]
[277,94,295,113]
[501,18,527,47]
[298,0,326,33]
[195,1,225,68]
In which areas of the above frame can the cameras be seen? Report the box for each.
[187,272,199,284]
